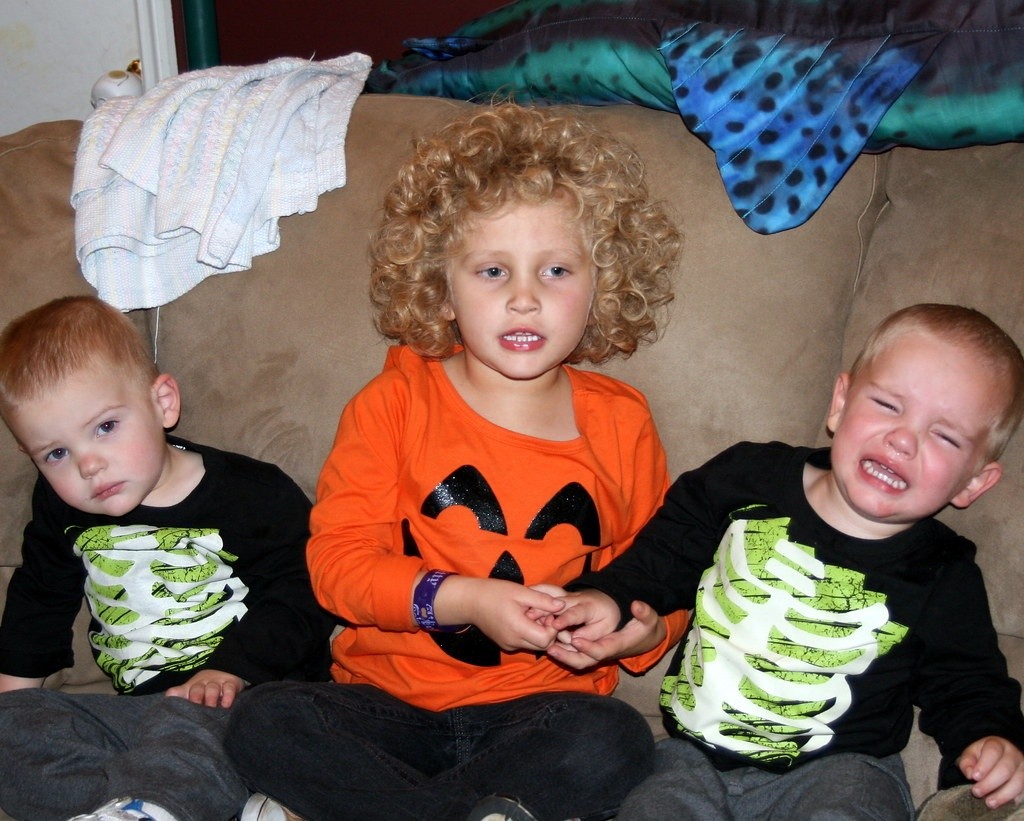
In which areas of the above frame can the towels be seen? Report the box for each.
[68,51,374,313]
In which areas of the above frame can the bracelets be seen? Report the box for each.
[413,569,471,635]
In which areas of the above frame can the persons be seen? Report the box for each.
[522,303,1024,821]
[225,100,689,821]
[0,296,333,821]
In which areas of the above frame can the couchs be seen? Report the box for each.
[0,93,1024,821]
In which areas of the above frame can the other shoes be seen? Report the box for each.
[466,792,545,821]
[240,793,307,821]
[67,795,179,821]
